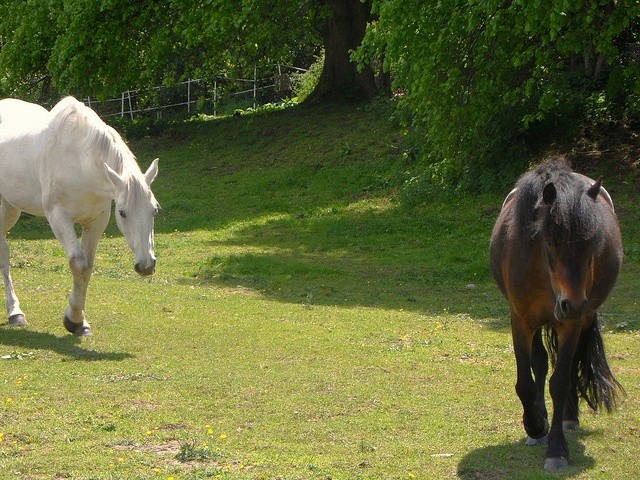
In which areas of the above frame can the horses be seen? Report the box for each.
[0,96,161,338]
[489,155,627,472]
